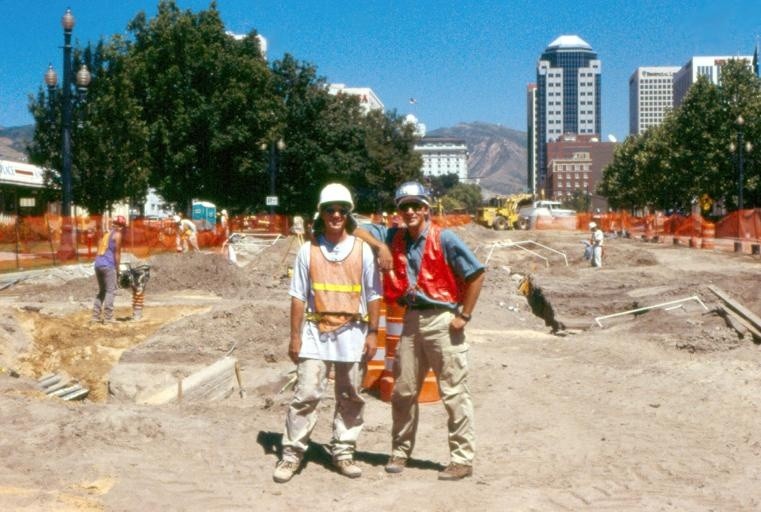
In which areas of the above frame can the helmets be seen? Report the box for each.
[394,181,430,202]
[113,215,126,225]
[173,215,181,223]
[317,182,354,212]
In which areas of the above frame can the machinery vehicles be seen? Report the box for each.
[476,193,533,231]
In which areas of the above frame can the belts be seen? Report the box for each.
[406,304,449,311]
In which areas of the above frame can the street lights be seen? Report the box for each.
[259,113,285,231]
[729,115,752,237]
[45,8,90,259]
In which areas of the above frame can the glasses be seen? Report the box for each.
[399,203,423,212]
[324,207,351,216]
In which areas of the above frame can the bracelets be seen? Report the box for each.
[458,312,472,321]
[368,328,379,333]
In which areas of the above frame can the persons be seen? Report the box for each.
[271,181,384,483]
[587,221,604,268]
[172,213,201,254]
[220,208,230,238]
[349,180,491,481]
[90,214,127,323]
[293,211,306,245]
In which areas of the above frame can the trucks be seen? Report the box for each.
[533,200,576,230]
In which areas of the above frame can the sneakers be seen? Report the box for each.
[384,453,408,473]
[332,457,364,479]
[270,458,303,483]
[436,462,475,481]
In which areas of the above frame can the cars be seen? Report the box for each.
[148,216,161,221]
